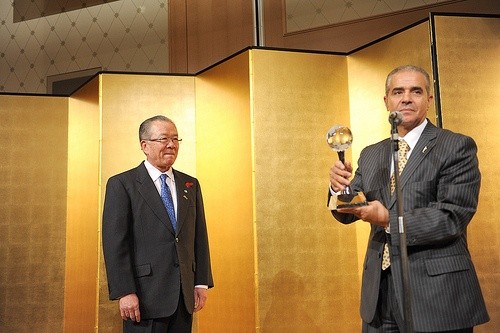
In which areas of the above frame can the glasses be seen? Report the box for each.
[140,135,183,146]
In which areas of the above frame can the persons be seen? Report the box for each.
[102,115,215,333]
[327,65,491,333]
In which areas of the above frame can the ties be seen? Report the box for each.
[160,174,177,232]
[381,139,410,271]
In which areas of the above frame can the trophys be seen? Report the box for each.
[326,124,370,211]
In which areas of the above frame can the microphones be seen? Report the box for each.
[388,111,403,126]
